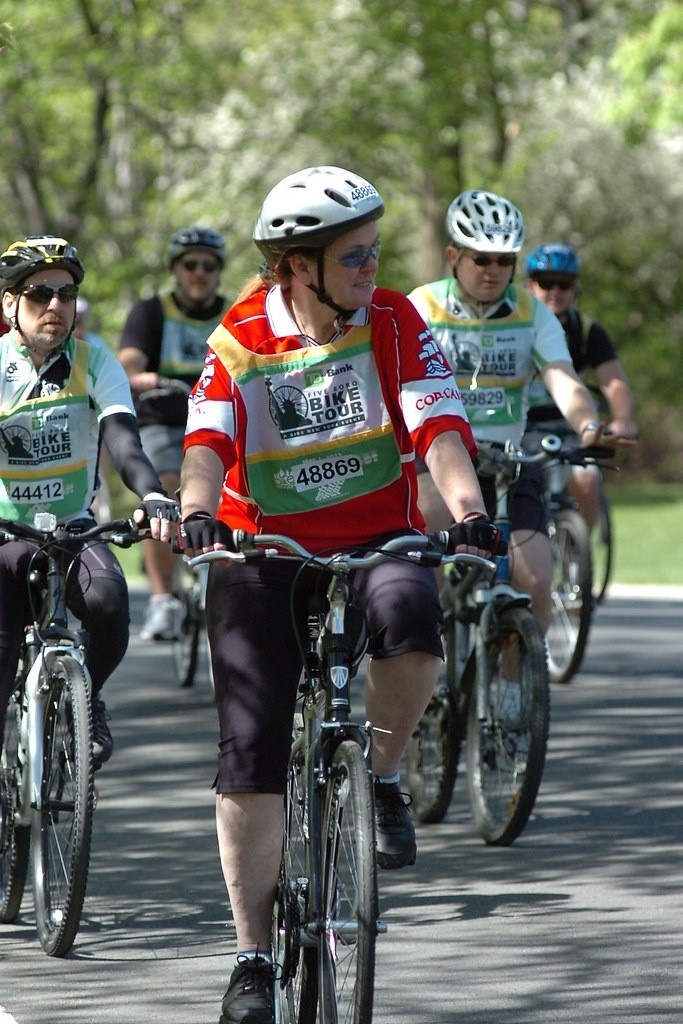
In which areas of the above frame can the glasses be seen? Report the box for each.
[532,275,573,290]
[177,259,218,272]
[313,240,382,268]
[14,283,78,304]
[457,247,515,266]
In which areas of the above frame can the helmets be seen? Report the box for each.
[0,236,84,301]
[166,226,226,270]
[446,188,525,254]
[254,165,384,267]
[528,241,577,277]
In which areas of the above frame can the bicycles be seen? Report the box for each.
[170,526,509,1024]
[0,516,172,957]
[399,432,622,847]
[543,425,634,686]
[149,492,209,690]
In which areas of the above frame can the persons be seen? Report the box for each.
[180,165,498,1024]
[120,227,233,639]
[0,235,181,762]
[526,243,638,594]
[405,190,639,764]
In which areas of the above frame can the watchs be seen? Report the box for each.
[582,423,598,435]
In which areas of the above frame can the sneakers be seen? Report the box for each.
[63,693,114,763]
[487,698,531,774]
[222,943,285,1024]
[366,771,417,871]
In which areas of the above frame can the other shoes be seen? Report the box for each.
[138,595,183,644]
